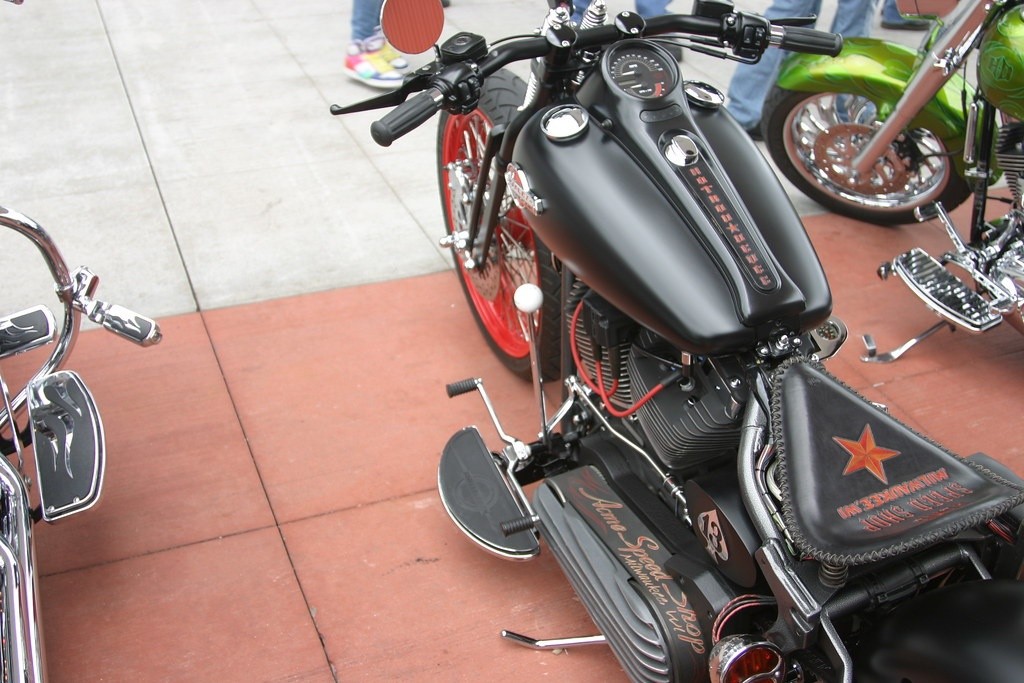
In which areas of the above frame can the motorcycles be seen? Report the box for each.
[0,202,168,682]
[327,0,1024,682]
[766,0,1023,365]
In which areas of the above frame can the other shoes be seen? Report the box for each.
[743,120,768,142]
[880,17,930,33]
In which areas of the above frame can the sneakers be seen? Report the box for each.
[369,25,412,77]
[343,36,406,89]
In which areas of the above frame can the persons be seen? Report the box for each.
[561,0,683,65]
[726,0,880,144]
[343,1,409,89]
[877,1,932,31]
